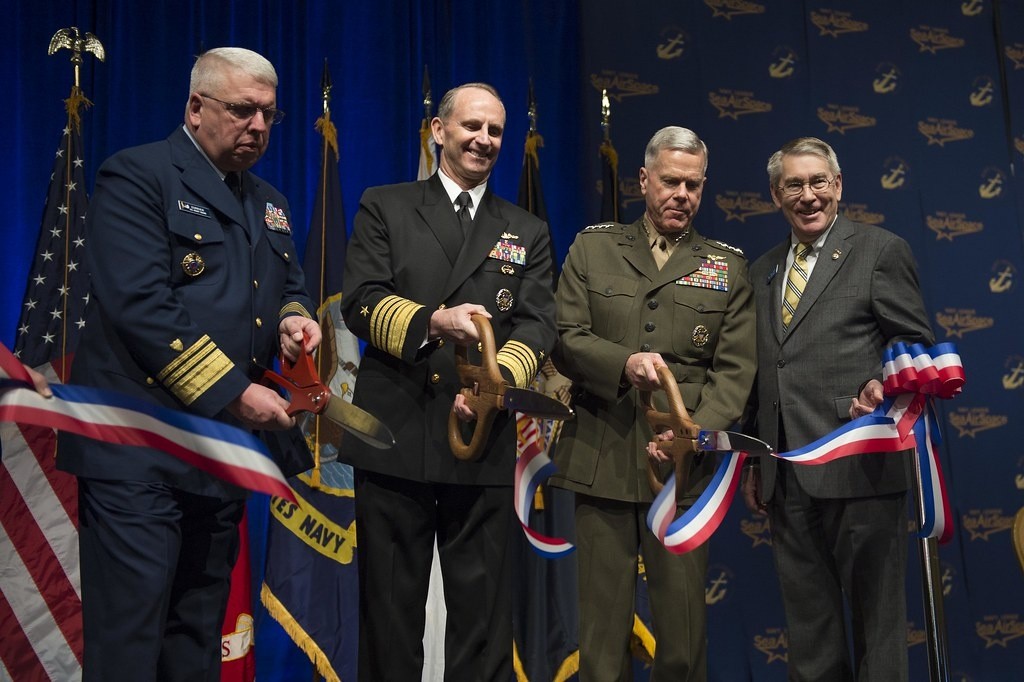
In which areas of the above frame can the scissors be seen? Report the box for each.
[261,329,398,451]
[445,313,579,459]
[636,365,773,492]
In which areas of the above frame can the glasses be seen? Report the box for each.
[200,93,285,125]
[776,176,836,196]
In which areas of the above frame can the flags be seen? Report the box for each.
[598,143,655,667]
[0,87,85,682]
[259,107,358,681]
[509,134,580,682]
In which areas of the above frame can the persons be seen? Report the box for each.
[55,46,322,682]
[740,137,937,679]
[0,362,51,408]
[547,127,757,682]
[339,83,557,682]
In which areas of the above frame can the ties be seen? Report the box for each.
[458,191,472,240]
[653,237,667,272]
[782,241,814,337]
[224,172,244,211]
